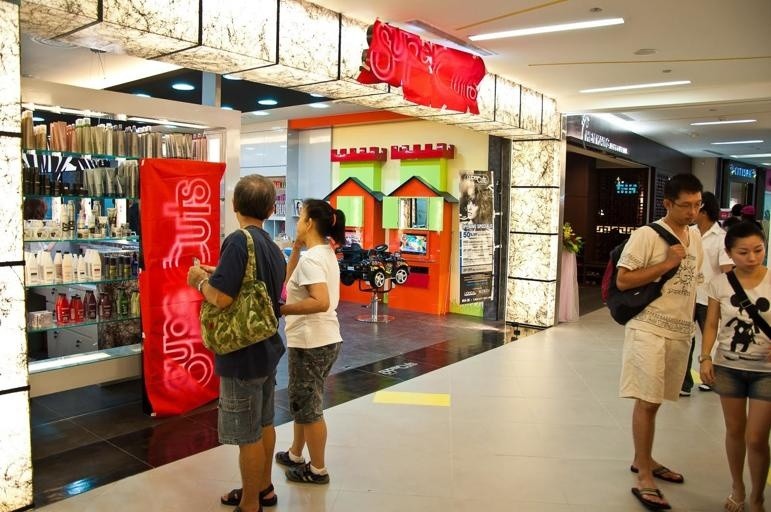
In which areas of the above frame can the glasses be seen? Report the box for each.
[670,199,704,210]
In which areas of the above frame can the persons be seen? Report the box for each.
[185,174,292,511]
[276,198,345,484]
[616,173,706,509]
[680,190,737,396]
[721,203,769,247]
[466,190,493,224]
[697,218,771,512]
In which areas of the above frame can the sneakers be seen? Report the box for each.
[679,390,691,397]
[275,452,306,467]
[287,464,332,484]
[698,384,712,391]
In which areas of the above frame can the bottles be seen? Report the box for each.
[60,198,75,238]
[102,252,138,280]
[77,201,131,238]
[23,112,210,162]
[55,287,140,323]
[25,248,101,282]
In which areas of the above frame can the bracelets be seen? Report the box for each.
[698,355,713,363]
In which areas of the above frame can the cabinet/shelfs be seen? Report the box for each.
[262,176,286,241]
[21,75,243,399]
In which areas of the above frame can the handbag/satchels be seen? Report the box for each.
[200,278,277,356]
[605,238,662,325]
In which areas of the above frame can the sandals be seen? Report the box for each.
[222,485,276,507]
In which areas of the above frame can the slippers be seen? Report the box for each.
[726,494,744,511]
[632,455,685,483]
[631,487,672,510]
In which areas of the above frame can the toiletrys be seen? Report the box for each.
[24,248,140,286]
[20,106,210,332]
[23,110,209,240]
[55,287,140,325]
